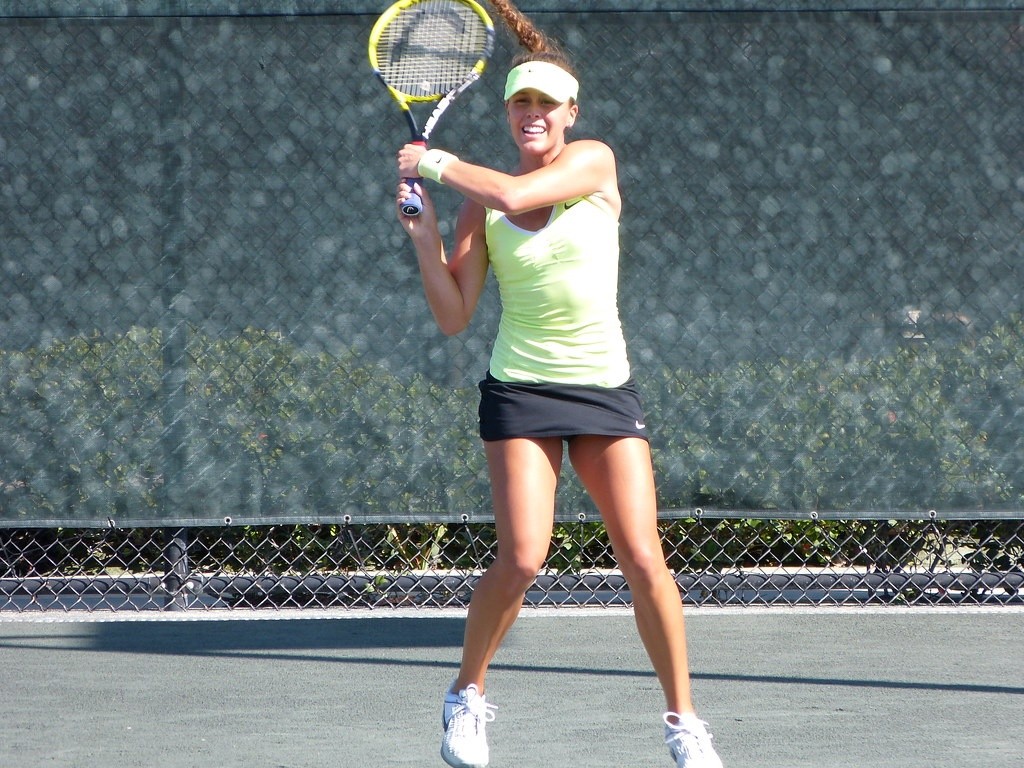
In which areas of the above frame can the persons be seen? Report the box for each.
[397,0,726,768]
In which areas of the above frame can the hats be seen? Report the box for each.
[503,61,580,104]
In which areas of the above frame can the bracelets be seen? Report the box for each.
[418,148,459,184]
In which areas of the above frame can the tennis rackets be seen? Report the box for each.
[368,0,496,217]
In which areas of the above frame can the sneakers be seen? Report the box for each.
[662,711,723,768]
[441,678,500,768]
